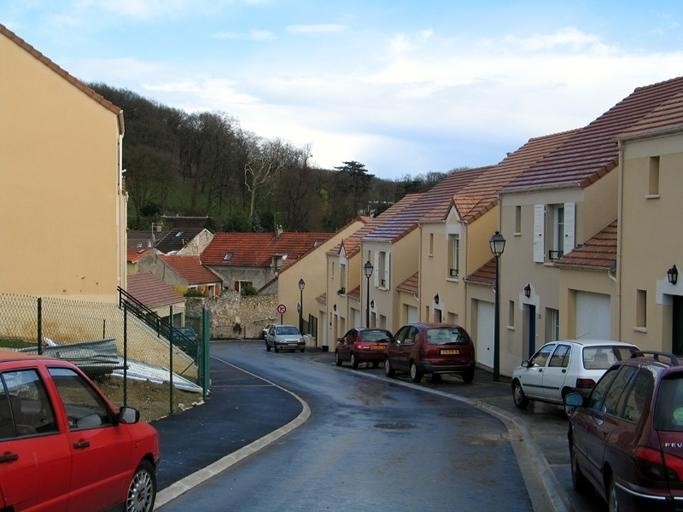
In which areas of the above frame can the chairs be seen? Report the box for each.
[591,352,610,369]
[403,331,451,343]
[0,394,33,435]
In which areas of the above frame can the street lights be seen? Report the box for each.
[489,231,506,381]
[299,279,305,334]
[364,260,373,328]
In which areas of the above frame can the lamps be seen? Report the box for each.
[371,300,374,308]
[524,284,530,298]
[667,264,678,285]
[334,304,336,311]
[434,294,439,304]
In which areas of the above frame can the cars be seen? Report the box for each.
[263,323,305,353]
[511,339,683,512]
[0,352,160,512]
[335,323,475,383]
